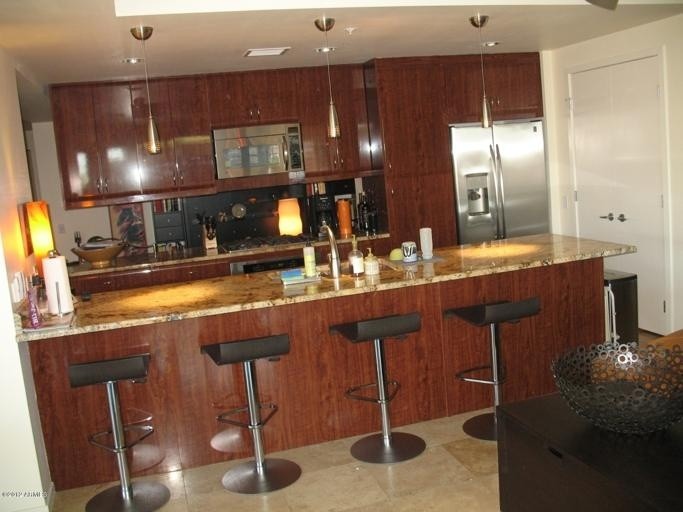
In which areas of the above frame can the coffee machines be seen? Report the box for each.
[306,181,338,238]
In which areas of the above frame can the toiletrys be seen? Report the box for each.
[279,269,302,280]
[348,234,364,277]
[364,247,379,276]
[304,238,316,277]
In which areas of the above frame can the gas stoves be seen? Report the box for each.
[220,234,315,253]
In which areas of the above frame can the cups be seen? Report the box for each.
[402,261,418,274]
[423,262,435,279]
[401,241,417,264]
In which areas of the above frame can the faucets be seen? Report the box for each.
[320,225,342,279]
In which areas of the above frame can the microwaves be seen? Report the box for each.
[214,123,303,180]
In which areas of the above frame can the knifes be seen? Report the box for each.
[203,214,217,238]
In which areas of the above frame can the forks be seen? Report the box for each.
[74,231,82,264]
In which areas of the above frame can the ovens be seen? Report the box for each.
[448,117,551,245]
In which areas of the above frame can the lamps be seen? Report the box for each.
[314,17,342,138]
[18,199,54,278]
[277,198,304,237]
[132,26,162,154]
[469,15,493,128]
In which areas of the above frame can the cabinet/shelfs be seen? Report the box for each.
[319,237,391,264]
[50,78,141,212]
[300,64,374,184]
[151,261,230,283]
[211,69,301,128]
[72,269,154,295]
[363,54,457,252]
[131,74,217,203]
[446,52,544,122]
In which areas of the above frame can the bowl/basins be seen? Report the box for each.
[69,239,126,267]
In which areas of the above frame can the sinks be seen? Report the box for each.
[322,261,393,276]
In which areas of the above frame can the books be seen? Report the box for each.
[280,267,305,282]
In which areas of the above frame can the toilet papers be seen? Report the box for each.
[40,256,75,316]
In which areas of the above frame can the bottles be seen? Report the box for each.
[420,227,434,261]
[356,188,376,232]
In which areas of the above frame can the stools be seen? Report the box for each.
[69,355,170,511]
[331,311,425,465]
[201,333,300,495]
[445,297,541,442]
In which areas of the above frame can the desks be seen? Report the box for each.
[497,383,682,510]
